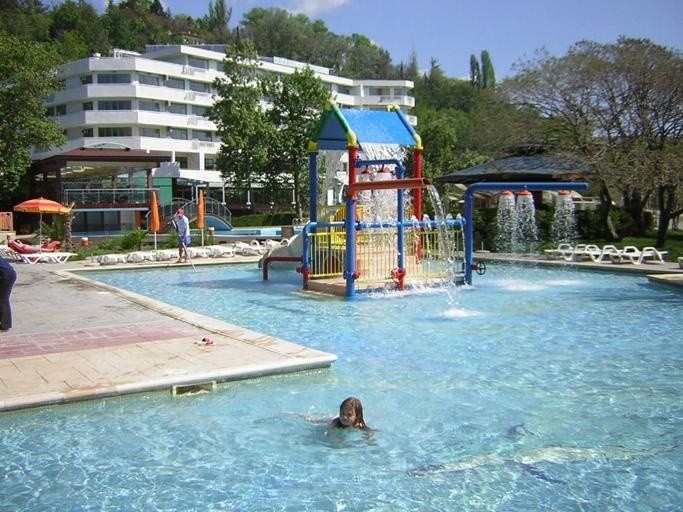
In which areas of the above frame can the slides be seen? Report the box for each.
[259,212,333,269]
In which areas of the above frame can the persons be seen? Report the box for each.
[0,256,16,330]
[172,207,191,264]
[7,234,44,248]
[281,397,379,448]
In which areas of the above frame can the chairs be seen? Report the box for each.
[94,234,297,267]
[0,242,76,265]
[539,241,667,266]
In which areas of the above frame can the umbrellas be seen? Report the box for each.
[13,196,69,254]
[198,189,205,247]
[151,191,160,251]
